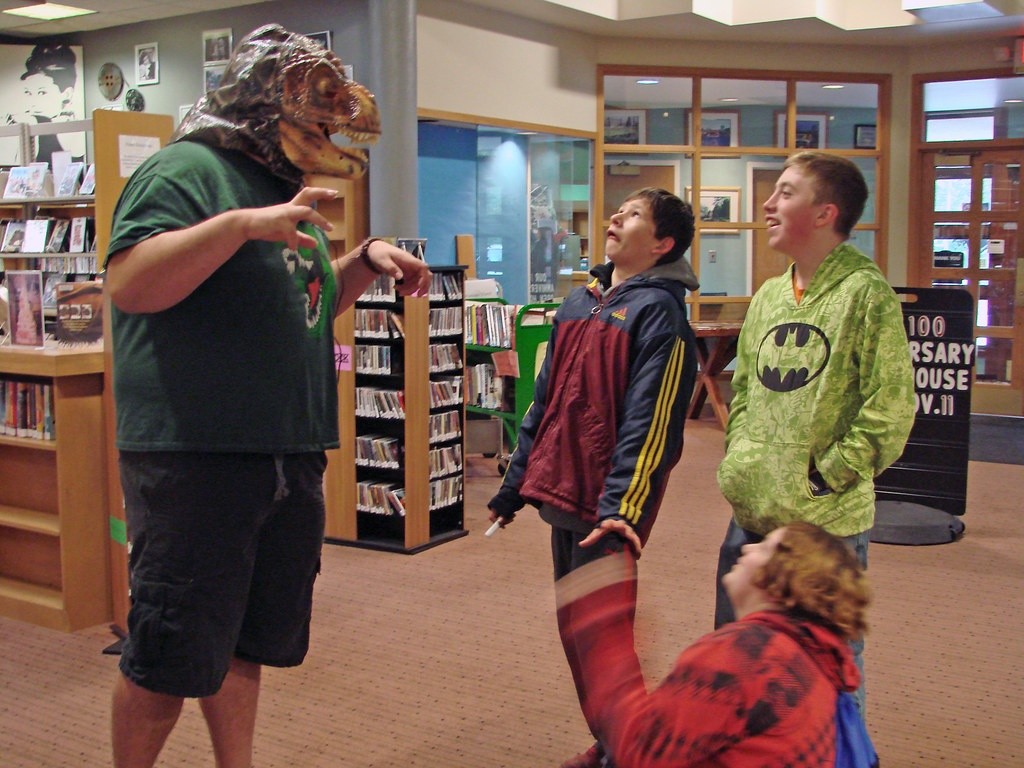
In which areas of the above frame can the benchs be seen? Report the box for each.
[346,265,470,555]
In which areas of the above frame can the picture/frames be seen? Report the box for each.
[684,185,740,235]
[853,124,879,149]
[773,109,829,158]
[684,107,742,159]
[134,23,354,129]
[602,108,648,157]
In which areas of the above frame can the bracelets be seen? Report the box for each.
[361,237,385,275]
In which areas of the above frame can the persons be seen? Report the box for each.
[560,520,872,768]
[489,186,700,768]
[715,152,914,723]
[103,26,430,768]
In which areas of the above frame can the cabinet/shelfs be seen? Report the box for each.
[462,298,562,461]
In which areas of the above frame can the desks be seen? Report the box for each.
[687,321,743,432]
[1,108,176,633]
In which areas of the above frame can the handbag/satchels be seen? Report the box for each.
[833,690,879,768]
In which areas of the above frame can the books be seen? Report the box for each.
[0,381,56,440]
[356,274,558,516]
